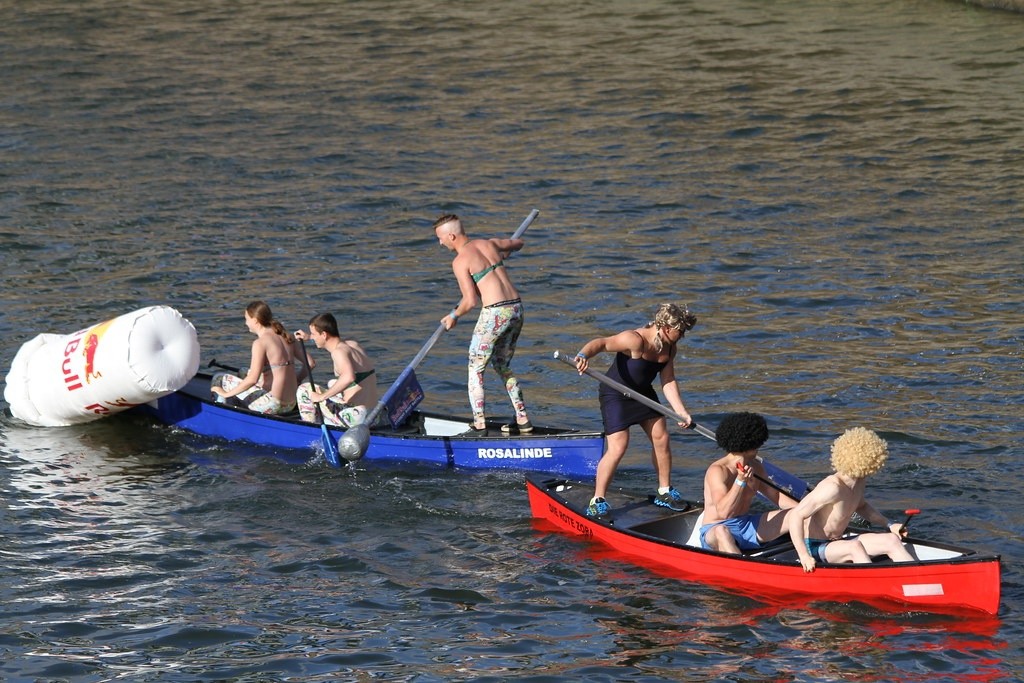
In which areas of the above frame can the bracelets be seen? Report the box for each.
[887,521,894,531]
[578,352,588,359]
[450,308,457,320]
[735,479,746,487]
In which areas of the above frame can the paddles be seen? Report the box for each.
[294,330,343,468]
[735,461,801,505]
[898,508,921,535]
[208,358,250,380]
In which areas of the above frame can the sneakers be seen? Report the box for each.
[654,486,690,511]
[587,496,614,525]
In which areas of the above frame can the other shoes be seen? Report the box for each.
[458,427,488,437]
[501,419,533,432]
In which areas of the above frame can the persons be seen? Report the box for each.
[210,300,315,415]
[575,303,697,524]
[699,411,799,556]
[295,312,377,428]
[789,427,915,572]
[433,214,533,436]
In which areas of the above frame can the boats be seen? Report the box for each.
[145,372,603,480]
[522,473,1001,615]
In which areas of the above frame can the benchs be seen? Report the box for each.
[279,411,302,420]
[378,423,419,434]
[746,539,796,559]
